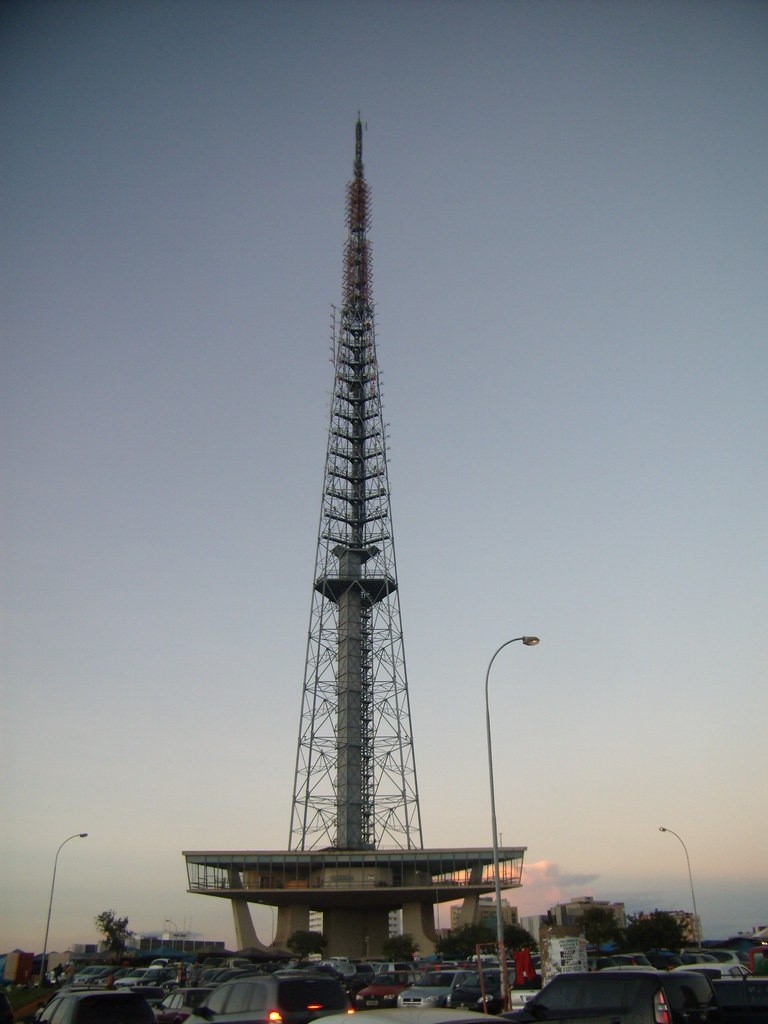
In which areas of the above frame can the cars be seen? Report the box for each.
[0,990,15,1024]
[1,927,768,1024]
[308,1006,519,1023]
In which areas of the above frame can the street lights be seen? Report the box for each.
[38,832,90,990]
[484,634,540,1010]
[659,826,702,953]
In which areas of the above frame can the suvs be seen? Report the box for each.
[24,989,159,1024]
[181,968,356,1024]
[500,969,727,1024]
